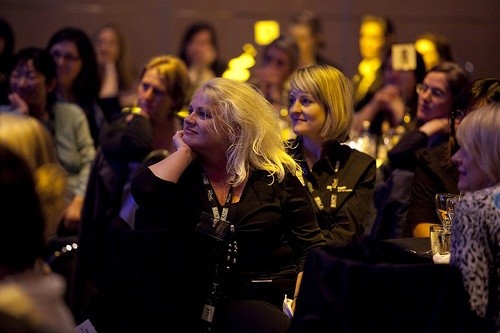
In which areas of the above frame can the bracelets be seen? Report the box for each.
[293,295,297,300]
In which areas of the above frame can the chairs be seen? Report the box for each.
[287,248,461,332]
[221,299,291,332]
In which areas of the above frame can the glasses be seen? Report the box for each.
[415,83,444,100]
[450,109,465,125]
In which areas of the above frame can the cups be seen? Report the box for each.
[429,225,451,255]
[435,194,456,225]
[446,198,455,221]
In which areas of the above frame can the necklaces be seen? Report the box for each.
[310,167,313,173]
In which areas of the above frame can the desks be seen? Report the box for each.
[376,235,432,261]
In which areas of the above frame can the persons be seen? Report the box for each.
[0,145,78,333]
[79,124,153,333]
[178,6,456,165]
[99,53,197,288]
[93,24,132,125]
[379,60,474,171]
[45,26,109,152]
[131,78,325,333]
[0,46,98,230]
[270,66,377,246]
[404,78,500,243]
[446,105,500,333]
